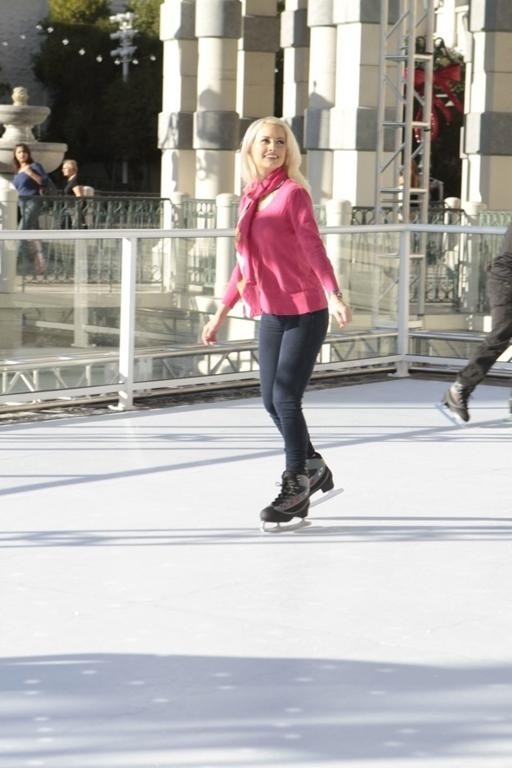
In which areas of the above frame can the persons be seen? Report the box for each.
[201,115,352,522]
[442,222,512,421]
[60,159,88,229]
[13,144,48,274]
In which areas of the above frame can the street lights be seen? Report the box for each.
[109,13,139,193]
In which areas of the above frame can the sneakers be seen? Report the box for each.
[261,472,309,524]
[443,375,475,422]
[304,453,334,498]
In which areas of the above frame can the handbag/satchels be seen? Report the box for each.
[39,177,57,211]
